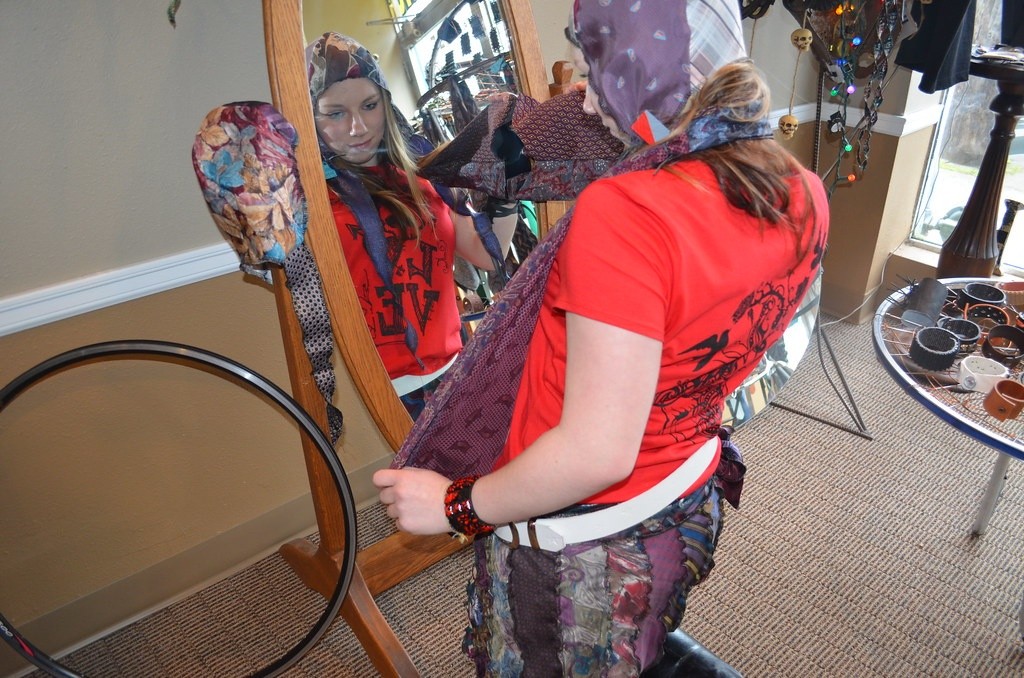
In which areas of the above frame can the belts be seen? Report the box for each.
[392,351,460,397]
[493,437,720,553]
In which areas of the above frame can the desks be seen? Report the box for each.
[874,276,1024,539]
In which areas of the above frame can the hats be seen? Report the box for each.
[192,100,305,266]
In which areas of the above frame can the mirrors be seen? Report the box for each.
[261,2,568,455]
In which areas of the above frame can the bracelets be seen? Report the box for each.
[446,479,494,536]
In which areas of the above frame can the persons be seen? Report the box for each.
[305,33,521,422]
[373,0,829,678]
